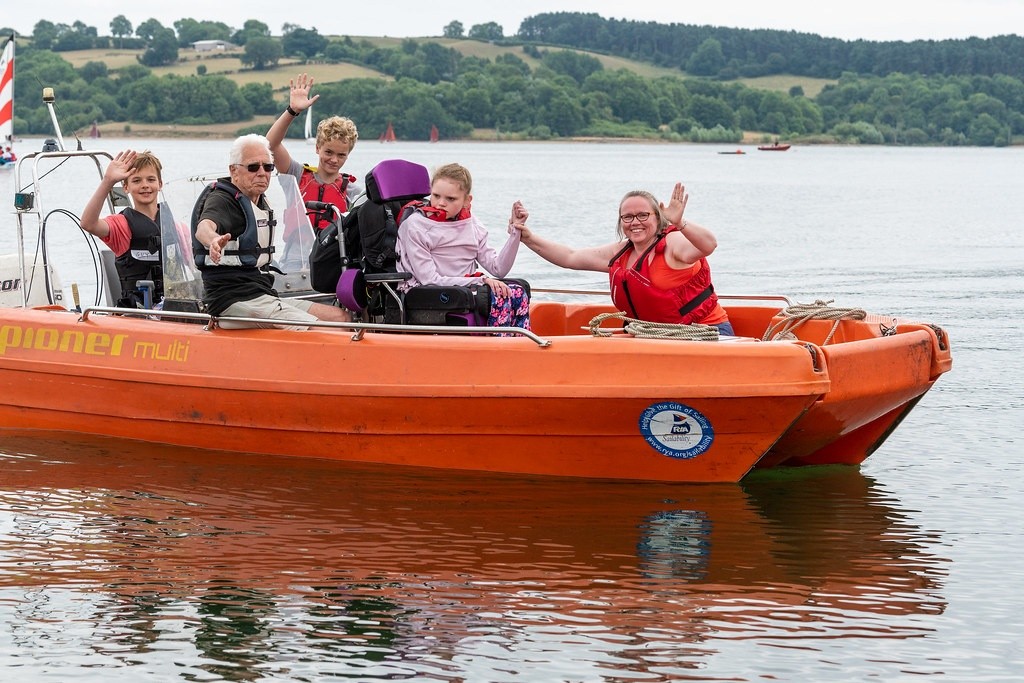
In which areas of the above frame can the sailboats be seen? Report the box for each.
[0,29,18,170]
[304,93,316,142]
[379,121,397,144]
[430,124,439,144]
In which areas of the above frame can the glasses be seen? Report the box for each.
[233,164,275,172]
[620,211,656,223]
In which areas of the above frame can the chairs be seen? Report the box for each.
[98,249,122,314]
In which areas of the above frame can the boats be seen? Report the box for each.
[759,144,792,151]
[1,88,953,482]
[718,150,745,154]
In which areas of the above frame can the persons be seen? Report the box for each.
[0,147,14,162]
[508,181,735,336]
[79,149,186,321]
[266,73,367,272]
[395,162,532,338]
[195,134,352,332]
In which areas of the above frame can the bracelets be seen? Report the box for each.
[287,105,299,116]
[678,218,686,230]
